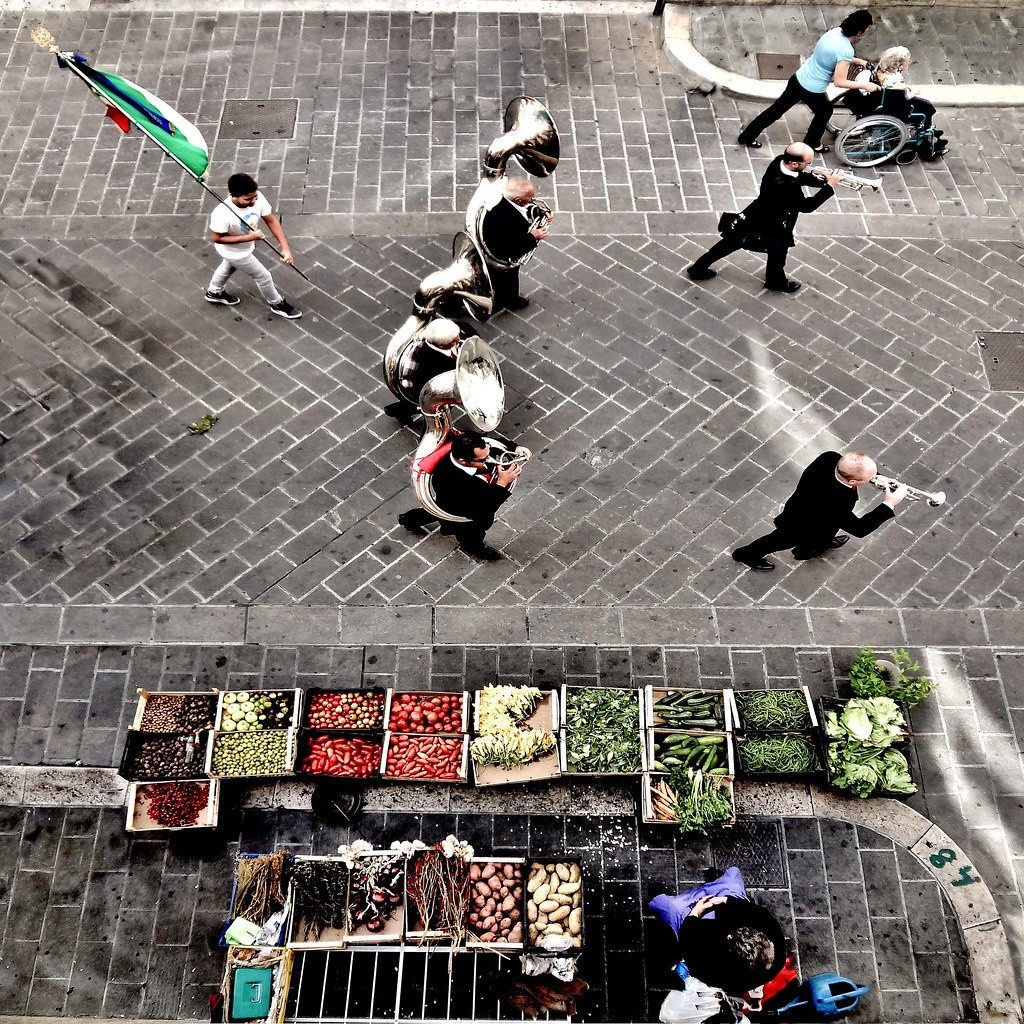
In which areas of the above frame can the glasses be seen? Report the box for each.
[464,456,489,463]
[789,161,812,167]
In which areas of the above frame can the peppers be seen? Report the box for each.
[407,843,469,932]
[142,780,210,827]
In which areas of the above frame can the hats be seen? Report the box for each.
[681,898,787,993]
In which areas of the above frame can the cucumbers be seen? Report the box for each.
[653,689,725,730]
[655,734,729,775]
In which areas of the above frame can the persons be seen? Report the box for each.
[396,430,533,562]
[474,175,554,322]
[204,172,303,319]
[731,450,908,571]
[383,317,481,428]
[736,10,882,152]
[874,44,949,153]
[647,864,787,997]
[687,141,845,292]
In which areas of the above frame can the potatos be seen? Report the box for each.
[469,862,523,943]
[527,862,582,948]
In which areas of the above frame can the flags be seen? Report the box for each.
[57,50,210,184]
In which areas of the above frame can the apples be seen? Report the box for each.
[307,691,385,729]
[388,694,463,734]
[221,692,263,731]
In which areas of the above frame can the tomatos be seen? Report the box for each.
[301,734,462,779]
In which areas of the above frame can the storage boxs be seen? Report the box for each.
[113,684,818,1024]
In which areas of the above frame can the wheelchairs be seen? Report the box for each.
[825,62,950,169]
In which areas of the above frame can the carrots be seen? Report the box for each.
[649,777,679,820]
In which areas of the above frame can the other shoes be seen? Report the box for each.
[705,867,724,883]
[462,543,504,560]
[384,404,414,426]
[935,139,948,149]
[493,297,528,313]
[647,883,664,900]
[397,513,428,535]
[934,130,944,137]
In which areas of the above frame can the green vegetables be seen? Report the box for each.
[668,767,734,837]
[567,687,643,772]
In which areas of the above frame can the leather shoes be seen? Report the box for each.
[731,548,774,570]
[689,269,717,280]
[738,138,762,148]
[827,535,849,550]
[814,144,830,153]
[771,282,801,293]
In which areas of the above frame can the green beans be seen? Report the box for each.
[736,689,819,772]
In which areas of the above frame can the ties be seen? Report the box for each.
[451,351,457,361]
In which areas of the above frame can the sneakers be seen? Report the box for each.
[204,290,240,305]
[271,298,302,319]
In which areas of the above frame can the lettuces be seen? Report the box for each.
[821,697,919,799]
